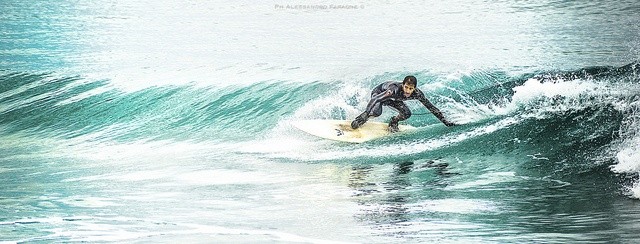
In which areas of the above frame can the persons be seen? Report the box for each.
[351,76,459,133]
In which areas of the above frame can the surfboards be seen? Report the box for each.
[290,119,416,143]
